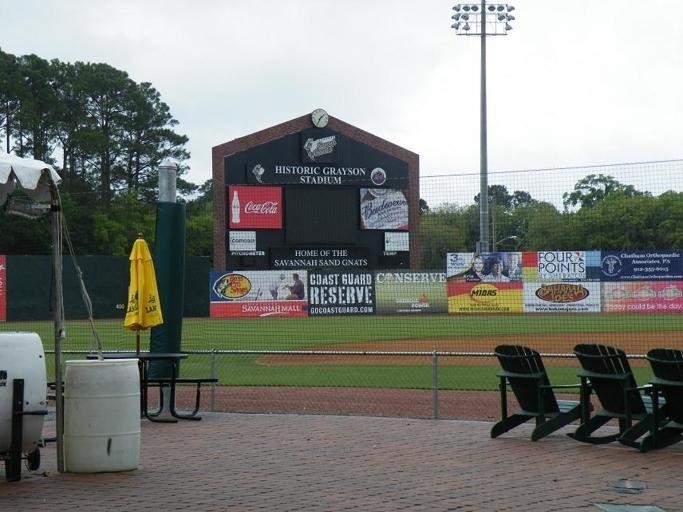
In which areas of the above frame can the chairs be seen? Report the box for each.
[489,340,682,455]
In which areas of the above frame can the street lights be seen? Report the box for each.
[451,1,516,252]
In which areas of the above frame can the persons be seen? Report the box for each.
[480,256,510,282]
[277,273,291,300]
[501,253,522,281]
[467,255,488,281]
[285,274,304,299]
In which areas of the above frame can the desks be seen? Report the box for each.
[87,353,188,424]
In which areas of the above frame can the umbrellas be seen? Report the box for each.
[123,231,164,351]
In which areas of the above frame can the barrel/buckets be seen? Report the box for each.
[63,359,142,474]
[0,332,48,455]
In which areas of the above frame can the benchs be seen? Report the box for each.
[143,377,220,423]
